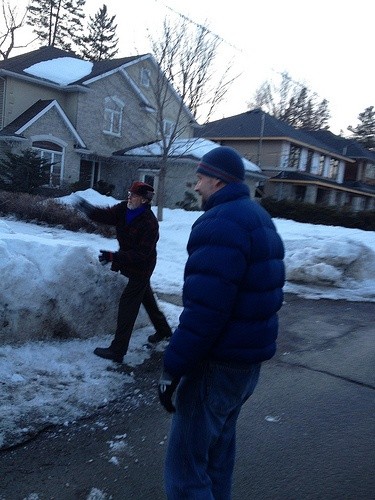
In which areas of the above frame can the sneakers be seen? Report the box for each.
[148,325,172,343]
[94,348,123,361]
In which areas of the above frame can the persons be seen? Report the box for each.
[73,181,173,363]
[158,147,286,499]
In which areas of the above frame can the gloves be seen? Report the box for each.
[159,374,176,410]
[99,250,115,266]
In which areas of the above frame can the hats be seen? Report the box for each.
[130,181,154,200]
[196,147,245,185]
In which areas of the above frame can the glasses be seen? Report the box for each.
[128,192,146,199]
